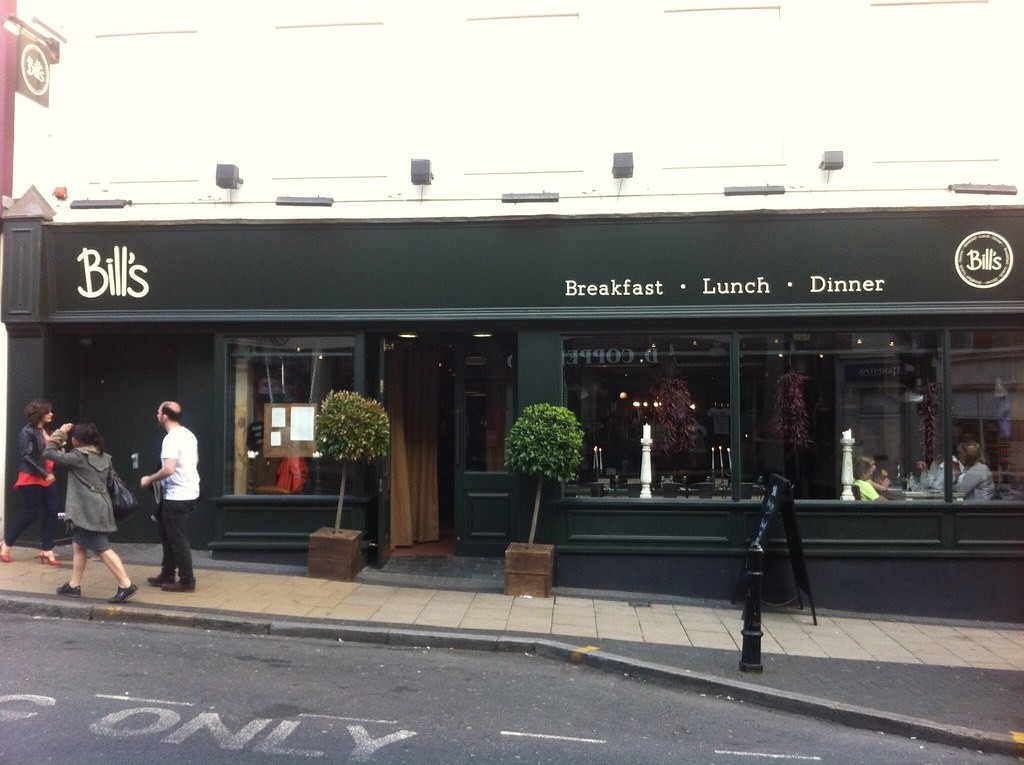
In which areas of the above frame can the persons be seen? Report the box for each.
[141,400,201,593]
[1,398,64,566]
[42,423,139,604]
[853,441,994,502]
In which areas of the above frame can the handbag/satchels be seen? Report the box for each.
[107,462,137,520]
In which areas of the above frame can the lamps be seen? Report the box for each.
[992,377,1009,399]
[611,152,634,179]
[215,163,243,191]
[818,151,844,170]
[412,158,433,186]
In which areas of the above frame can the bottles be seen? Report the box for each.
[906,471,917,491]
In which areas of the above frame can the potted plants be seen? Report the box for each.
[306,388,391,584]
[502,402,587,597]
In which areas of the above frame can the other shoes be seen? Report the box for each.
[108,581,137,603]
[161,581,194,593]
[57,581,81,597]
[147,568,176,584]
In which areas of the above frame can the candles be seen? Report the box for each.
[712,446,733,470]
[592,446,602,471]
[642,422,652,442]
[842,427,852,440]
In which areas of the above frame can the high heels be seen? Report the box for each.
[39,550,62,565]
[0,540,11,562]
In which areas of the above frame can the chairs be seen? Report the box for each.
[622,479,764,501]
[820,479,864,500]
